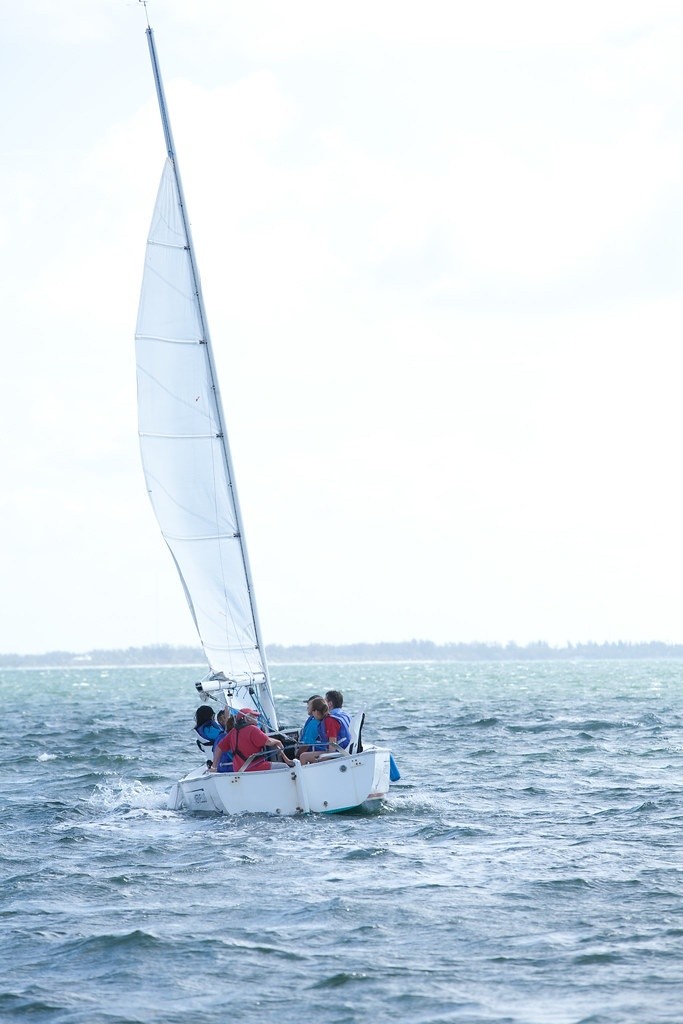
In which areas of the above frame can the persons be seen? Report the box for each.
[194,705,238,772]
[285,690,352,765]
[207,708,289,772]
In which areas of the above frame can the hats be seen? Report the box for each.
[237,708,260,719]
[303,695,321,703]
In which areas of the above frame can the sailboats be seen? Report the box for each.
[134,0,402,817]
